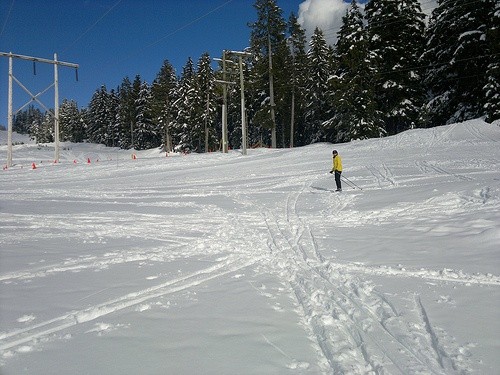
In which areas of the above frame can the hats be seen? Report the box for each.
[333,150,337,154]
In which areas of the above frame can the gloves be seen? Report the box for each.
[330,171,333,173]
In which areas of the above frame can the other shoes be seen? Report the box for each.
[336,189,342,191]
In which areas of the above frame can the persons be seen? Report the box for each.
[329,150,343,190]
